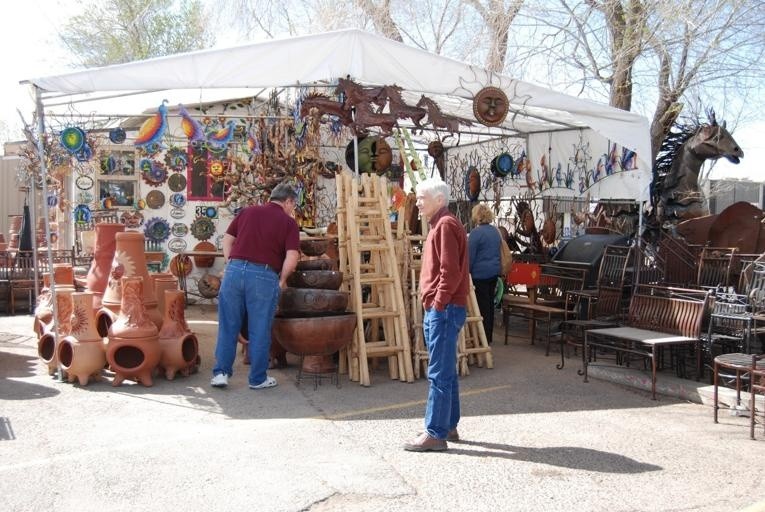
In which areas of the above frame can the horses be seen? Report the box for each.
[335,77,388,114]
[375,83,426,136]
[415,95,478,147]
[650,105,745,220]
[298,90,355,138]
[342,90,399,141]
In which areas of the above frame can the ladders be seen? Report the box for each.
[336,172,492,387]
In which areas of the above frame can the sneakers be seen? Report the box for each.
[210,373,229,386]
[248,376,278,389]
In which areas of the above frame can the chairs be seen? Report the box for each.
[0,207,123,316]
[499,241,765,443]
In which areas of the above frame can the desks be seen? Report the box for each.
[144,249,166,274]
[176,248,226,304]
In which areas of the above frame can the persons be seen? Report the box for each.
[210,182,301,390]
[402,177,469,452]
[467,202,502,343]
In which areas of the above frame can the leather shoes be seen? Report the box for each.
[417,426,460,442]
[402,431,448,452]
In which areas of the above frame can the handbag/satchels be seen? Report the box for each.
[494,226,513,275]
